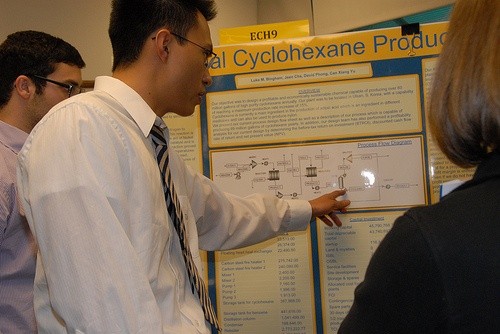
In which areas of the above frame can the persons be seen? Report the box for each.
[0,31,87,334]
[15,0,351,334]
[335,0,500,334]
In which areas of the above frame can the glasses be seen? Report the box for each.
[151,33,217,75]
[14,76,85,98]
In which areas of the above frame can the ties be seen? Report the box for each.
[149,122,221,334]
